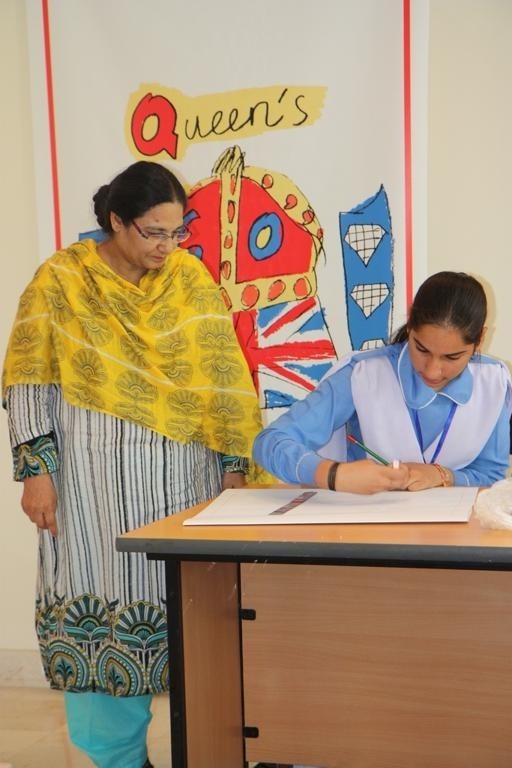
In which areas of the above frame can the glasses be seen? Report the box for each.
[130,219,192,243]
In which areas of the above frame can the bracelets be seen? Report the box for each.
[327,461,340,492]
[432,462,454,487]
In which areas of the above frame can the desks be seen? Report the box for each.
[117,485,511,768]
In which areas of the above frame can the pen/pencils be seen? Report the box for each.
[347,434,390,466]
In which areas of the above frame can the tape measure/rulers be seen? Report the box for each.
[269,492,317,515]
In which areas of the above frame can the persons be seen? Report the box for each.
[250,271,512,495]
[1,160,281,768]
